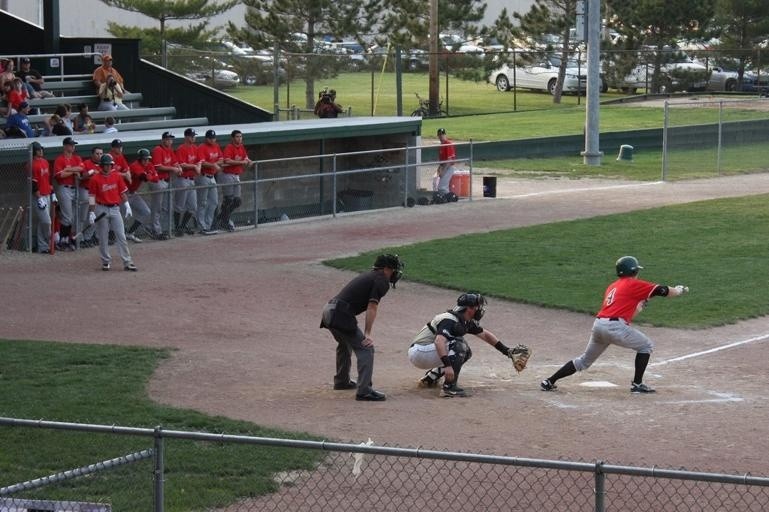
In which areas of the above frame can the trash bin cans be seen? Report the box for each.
[340,190,373,212]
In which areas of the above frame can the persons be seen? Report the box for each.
[89,155,137,270]
[27,128,253,254]
[93,56,130,112]
[407,291,529,398]
[540,256,689,394]
[1,57,55,139]
[102,116,118,134]
[437,128,455,195]
[314,90,344,118]
[40,102,97,136]
[320,255,404,402]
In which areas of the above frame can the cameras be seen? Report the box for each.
[111,80,116,85]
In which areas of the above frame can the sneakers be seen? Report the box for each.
[439,387,472,397]
[355,391,386,401]
[103,263,110,270]
[124,263,137,270]
[419,376,442,386]
[143,216,234,240]
[42,231,143,253]
[629,381,656,394]
[334,379,357,389]
[540,379,559,391]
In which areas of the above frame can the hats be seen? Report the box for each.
[185,128,197,136]
[63,137,78,145]
[112,139,124,147]
[163,131,175,138]
[22,57,30,63]
[205,130,217,139]
[104,55,113,61]
[437,128,445,135]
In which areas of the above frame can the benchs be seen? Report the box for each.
[0,80,209,138]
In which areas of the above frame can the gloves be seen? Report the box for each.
[675,285,685,296]
[37,197,47,209]
[51,193,58,205]
[637,300,647,313]
[88,211,96,224]
[124,201,133,218]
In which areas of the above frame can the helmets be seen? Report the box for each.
[453,290,487,321]
[136,149,152,160]
[30,141,44,155]
[616,256,643,276]
[100,154,115,167]
[372,253,405,288]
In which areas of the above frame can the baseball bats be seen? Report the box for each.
[2,206,29,251]
[50,202,56,255]
[72,213,106,240]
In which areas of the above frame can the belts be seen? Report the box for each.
[203,173,214,178]
[185,177,193,180]
[99,202,119,207]
[85,187,89,190]
[162,178,168,182]
[64,185,74,189]
[597,316,619,321]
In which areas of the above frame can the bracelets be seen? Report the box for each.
[440,356,451,367]
[495,341,509,355]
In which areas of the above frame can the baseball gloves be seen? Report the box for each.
[507,344,531,372]
